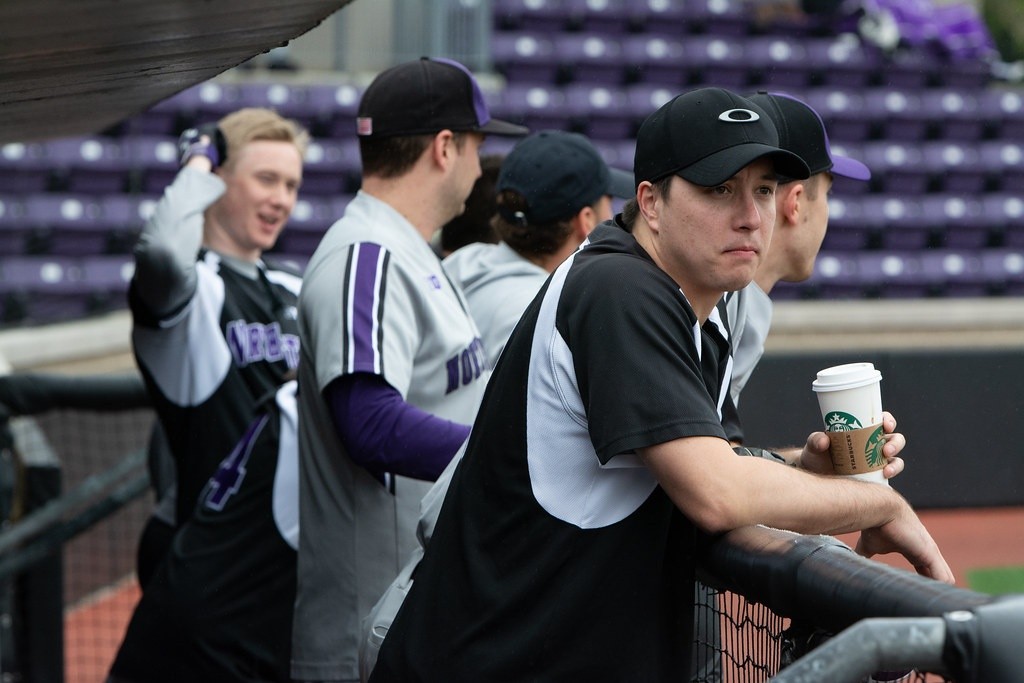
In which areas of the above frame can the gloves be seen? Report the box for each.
[176,120,228,168]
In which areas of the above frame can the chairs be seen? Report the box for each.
[1,1,1024,327]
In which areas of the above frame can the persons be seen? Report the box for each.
[367,83,956,683]
[104,54,872,683]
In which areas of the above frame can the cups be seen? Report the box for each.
[811,362,890,486]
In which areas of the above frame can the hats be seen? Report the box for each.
[353,56,530,138]
[744,90,872,181]
[491,130,637,221]
[633,87,811,187]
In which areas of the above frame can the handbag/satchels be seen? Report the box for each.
[168,498,295,648]
